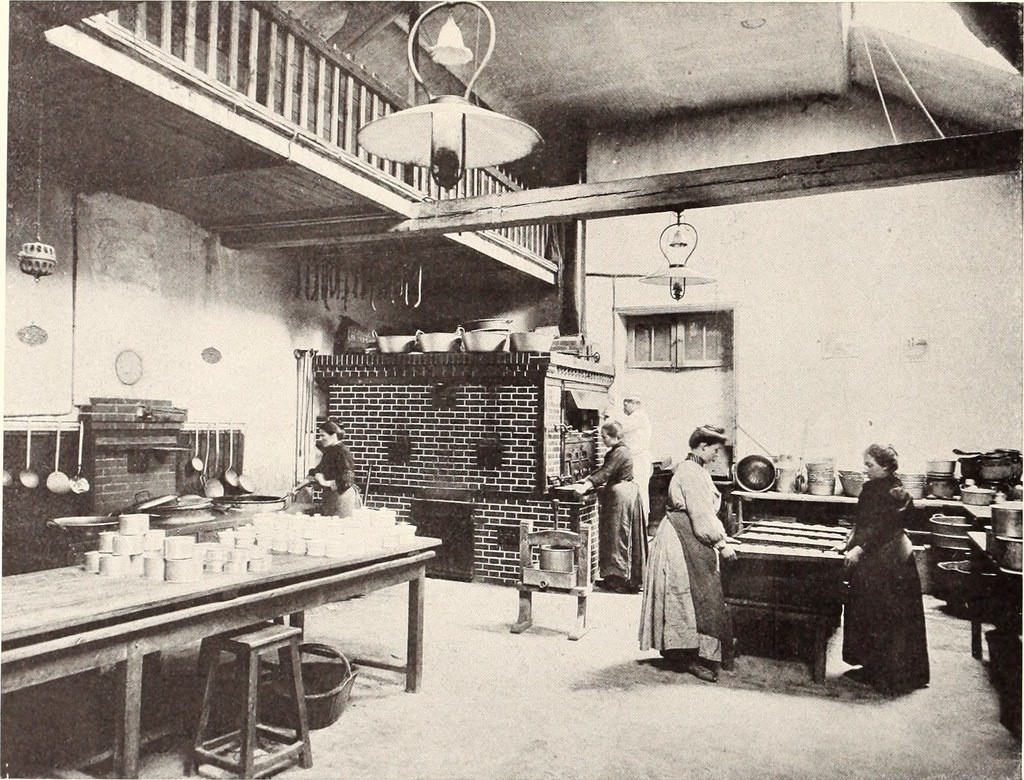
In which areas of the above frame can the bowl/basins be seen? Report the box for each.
[372,330,421,353]
[990,533,1023,570]
[838,470,869,497]
[928,460,955,473]
[983,526,993,552]
[901,474,925,499]
[417,327,466,352]
[462,332,509,351]
[991,502,1023,537]
[805,463,835,495]
[511,332,558,352]
[959,484,996,506]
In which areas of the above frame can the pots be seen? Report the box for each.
[731,455,780,493]
[952,448,1023,482]
[904,513,973,549]
[46,510,123,538]
[926,472,957,499]
[212,495,287,510]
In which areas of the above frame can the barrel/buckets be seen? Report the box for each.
[539,545,575,572]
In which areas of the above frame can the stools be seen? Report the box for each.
[183,621,314,780]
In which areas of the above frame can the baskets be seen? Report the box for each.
[274,644,361,731]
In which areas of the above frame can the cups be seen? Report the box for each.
[84,507,417,582]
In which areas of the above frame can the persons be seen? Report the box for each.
[831,444,931,694]
[287,419,365,600]
[638,424,741,682]
[574,420,648,593]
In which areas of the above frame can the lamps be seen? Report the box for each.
[639,208,718,301]
[355,1,547,192]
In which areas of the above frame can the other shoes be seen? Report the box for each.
[689,662,717,682]
[615,586,639,594]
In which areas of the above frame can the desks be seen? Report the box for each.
[967,531,1022,659]
[1,536,442,780]
[730,489,994,532]
